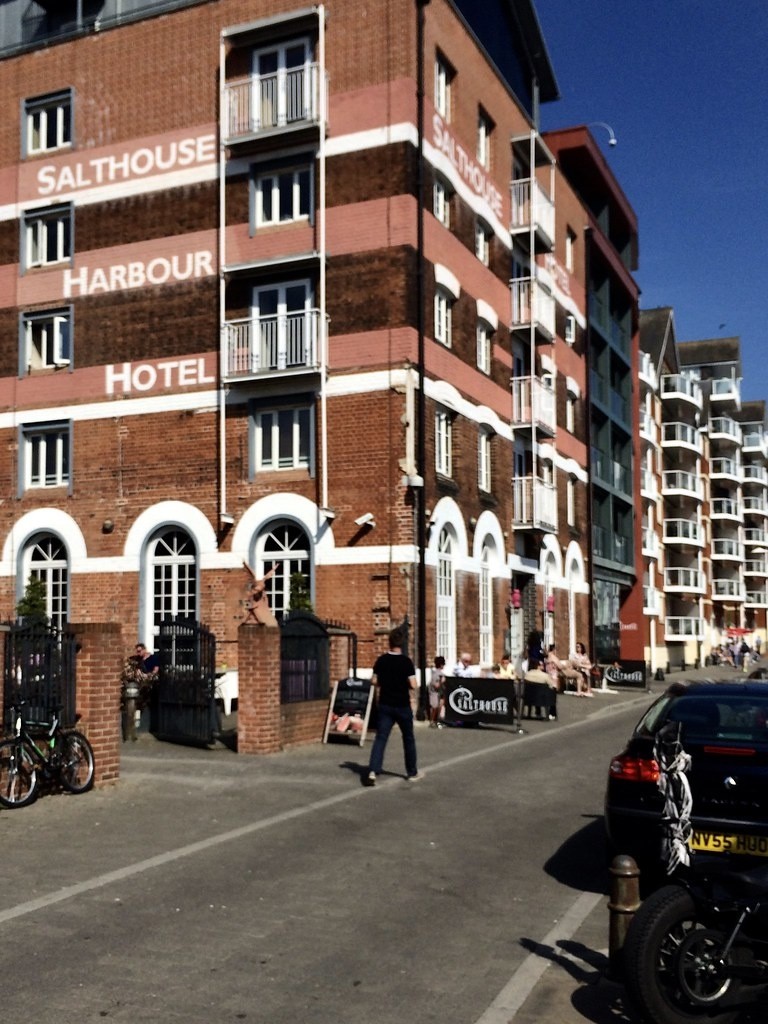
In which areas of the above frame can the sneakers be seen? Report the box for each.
[408,771,425,781]
[368,771,376,780]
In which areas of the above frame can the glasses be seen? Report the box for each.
[464,660,472,664]
[137,650,143,653]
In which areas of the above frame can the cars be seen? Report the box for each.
[747,668,768,684]
[604,676,768,864]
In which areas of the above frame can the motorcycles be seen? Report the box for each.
[624,867,768,1023]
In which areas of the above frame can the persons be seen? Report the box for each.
[712,634,762,673]
[524,624,548,672]
[548,643,584,697]
[453,653,473,677]
[487,664,500,679]
[498,654,524,719]
[429,657,446,728]
[136,644,159,679]
[368,630,424,786]
[572,642,601,690]
[521,649,560,691]
[523,660,558,720]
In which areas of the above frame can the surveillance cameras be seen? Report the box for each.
[356,513,374,526]
[430,515,436,526]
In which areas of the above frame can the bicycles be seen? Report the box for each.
[0,701,94,808]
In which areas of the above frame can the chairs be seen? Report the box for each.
[522,679,557,720]
[214,671,239,716]
